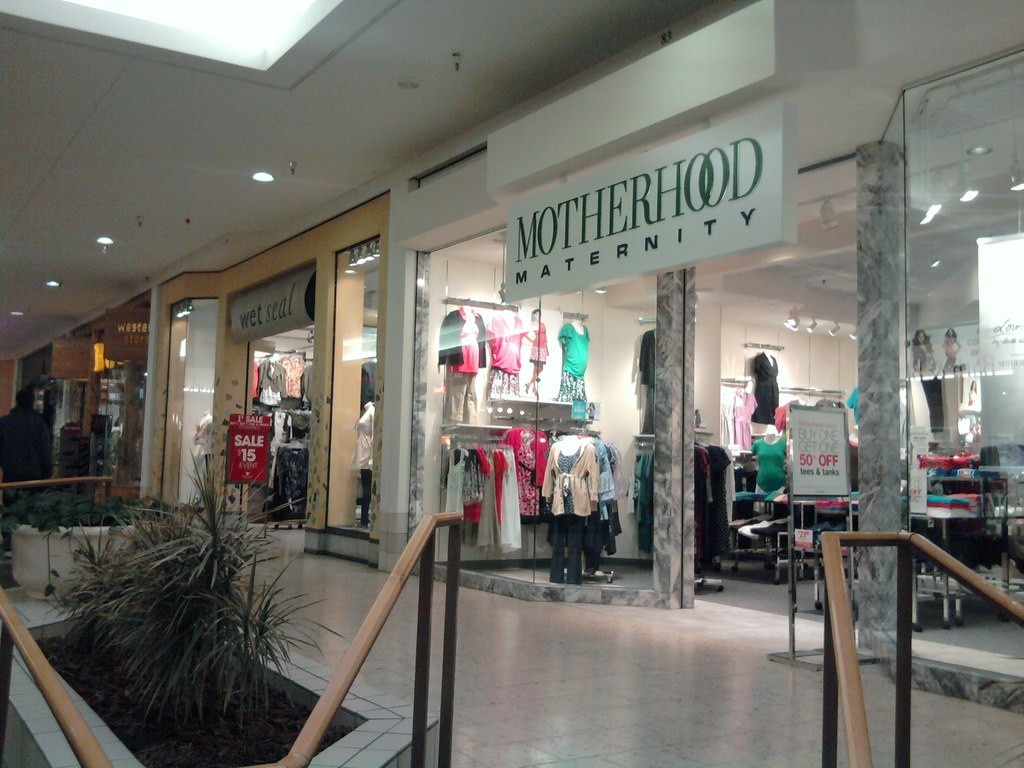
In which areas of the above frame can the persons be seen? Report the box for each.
[438,306,488,426]
[0,386,55,558]
[557,318,591,403]
[545,435,598,584]
[484,310,529,402]
[350,402,380,527]
[528,309,551,400]
[748,425,789,512]
[910,327,981,441]
